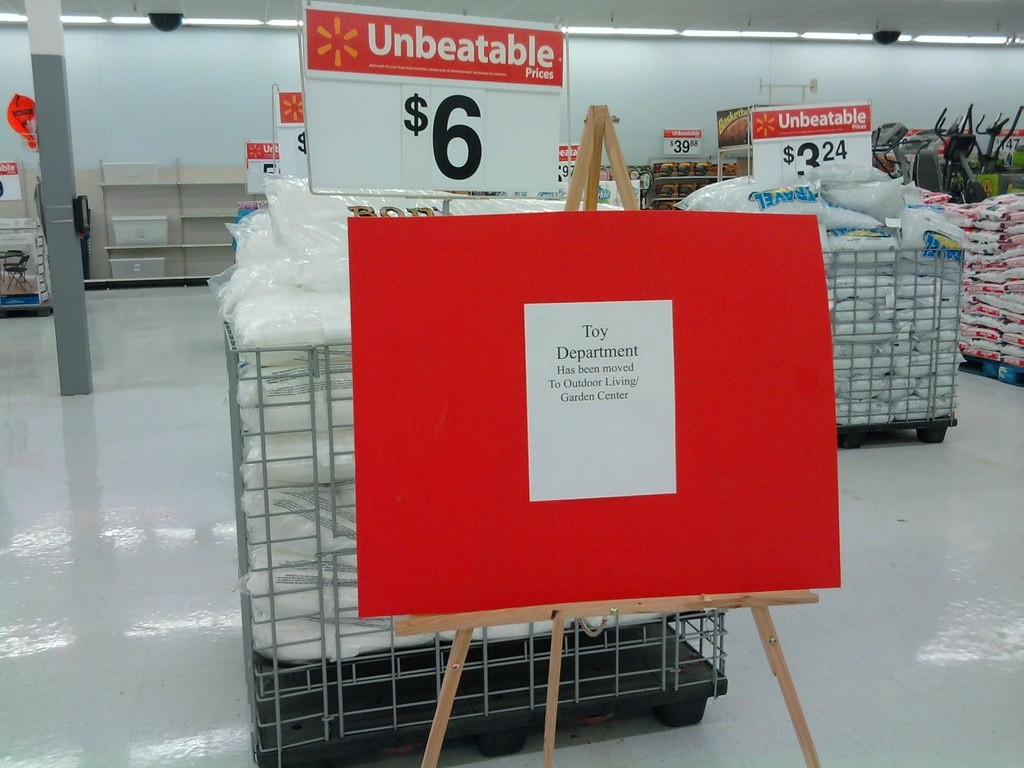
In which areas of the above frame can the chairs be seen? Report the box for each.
[5,255,33,291]
[3,250,25,285]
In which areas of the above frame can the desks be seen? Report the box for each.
[0,253,25,284]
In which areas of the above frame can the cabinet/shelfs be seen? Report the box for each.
[645,161,737,210]
[83,161,272,289]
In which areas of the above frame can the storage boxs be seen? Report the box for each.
[109,257,166,280]
[0,218,52,305]
[112,216,168,247]
